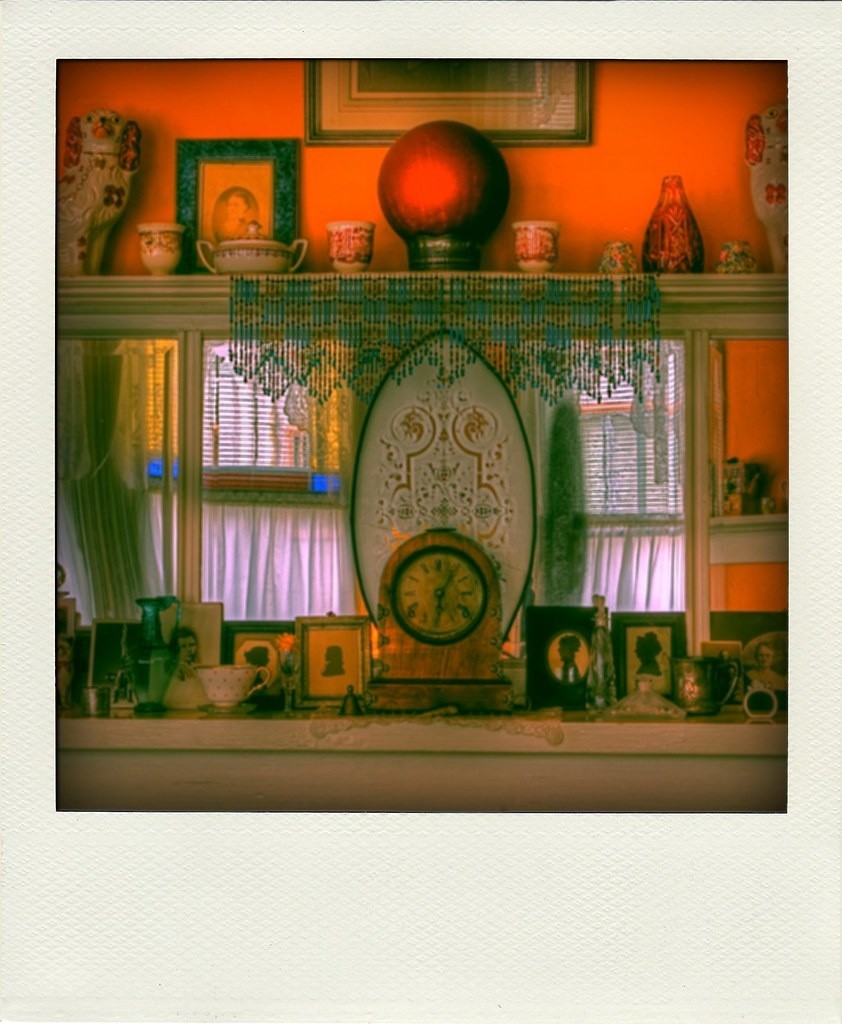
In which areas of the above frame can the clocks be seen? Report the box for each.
[367,527,514,710]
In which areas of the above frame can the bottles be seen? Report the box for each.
[745,102,788,273]
[642,175,704,274]
[122,595,181,713]
[584,613,616,711]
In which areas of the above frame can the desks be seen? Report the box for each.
[58,705,788,812]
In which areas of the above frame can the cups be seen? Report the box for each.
[714,239,760,274]
[136,222,184,276]
[599,241,638,274]
[512,220,560,273]
[194,665,271,708]
[671,656,740,716]
[327,222,375,272]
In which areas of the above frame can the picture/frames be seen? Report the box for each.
[303,60,593,145]
[219,621,296,710]
[177,137,297,275]
[611,611,688,705]
[296,615,370,706]
[526,606,608,709]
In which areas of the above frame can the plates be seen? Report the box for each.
[198,703,256,717]
